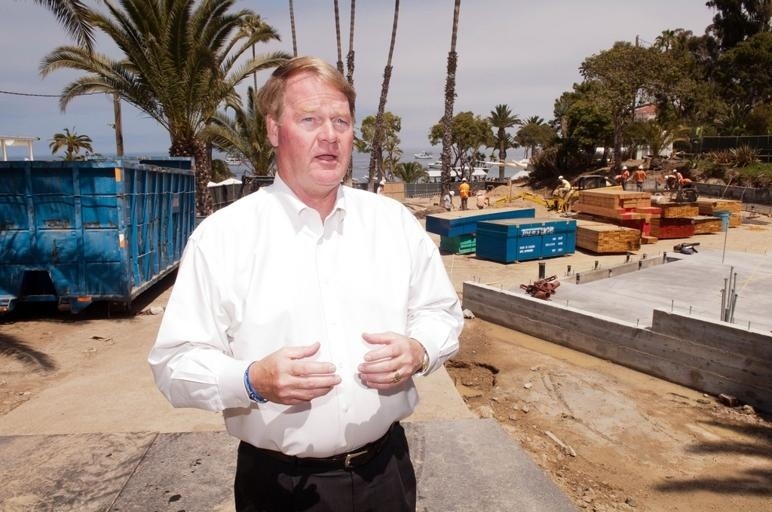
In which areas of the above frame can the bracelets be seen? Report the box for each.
[245,358,270,405]
[414,350,430,374]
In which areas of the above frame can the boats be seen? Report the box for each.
[224,155,241,165]
[428,160,442,169]
[414,152,432,159]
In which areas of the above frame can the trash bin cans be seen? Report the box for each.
[713,212,729,232]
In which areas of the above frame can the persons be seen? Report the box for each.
[458,177,471,211]
[663,174,676,189]
[147,55,467,510]
[657,171,670,192]
[678,178,693,189]
[442,190,457,213]
[610,174,622,186]
[671,168,684,190]
[631,163,647,192]
[621,165,631,190]
[557,175,571,200]
[375,180,385,195]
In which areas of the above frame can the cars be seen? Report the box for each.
[571,175,620,190]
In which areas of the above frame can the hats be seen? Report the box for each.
[449,191,455,197]
[557,176,563,181]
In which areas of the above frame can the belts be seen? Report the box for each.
[247,448,388,475]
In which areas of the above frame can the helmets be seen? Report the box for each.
[622,165,643,170]
[664,168,677,181]
[462,178,468,182]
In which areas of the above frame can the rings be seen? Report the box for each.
[391,369,401,383]
